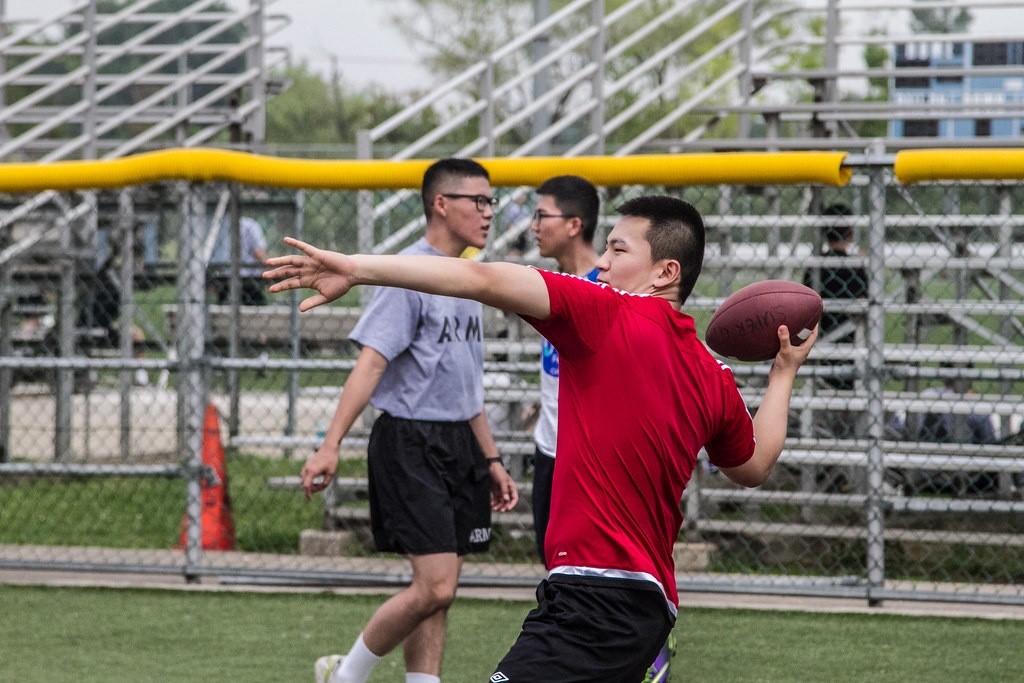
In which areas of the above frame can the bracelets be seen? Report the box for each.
[486,454,506,467]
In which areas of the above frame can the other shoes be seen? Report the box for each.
[314,654,343,682]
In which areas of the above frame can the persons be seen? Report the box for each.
[216,205,274,302]
[262,194,821,683]
[799,204,872,392]
[299,157,520,683]
[525,176,678,683]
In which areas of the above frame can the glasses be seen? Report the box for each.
[533,210,584,228]
[431,194,500,212]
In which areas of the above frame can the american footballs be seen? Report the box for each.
[704,278,824,362]
